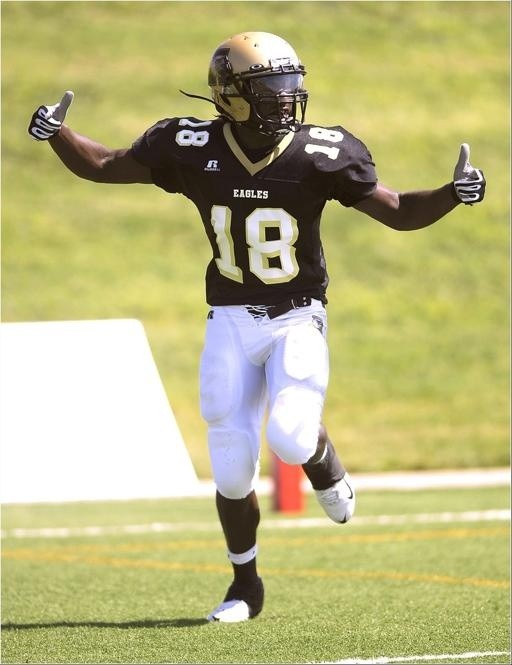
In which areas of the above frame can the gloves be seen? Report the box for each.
[453,143,485,204]
[28,91,73,141]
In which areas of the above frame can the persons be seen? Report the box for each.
[28,29,486,621]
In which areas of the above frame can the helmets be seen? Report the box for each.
[207,32,307,126]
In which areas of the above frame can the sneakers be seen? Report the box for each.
[210,579,263,623]
[301,442,355,524]
